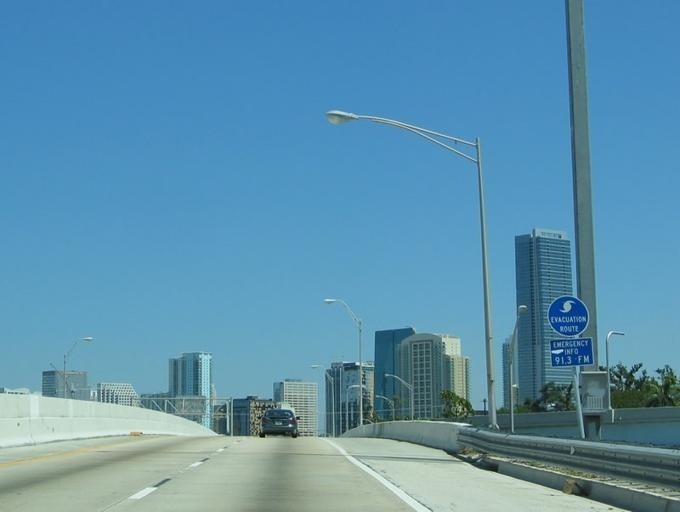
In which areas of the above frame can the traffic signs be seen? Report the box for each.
[547,294,595,368]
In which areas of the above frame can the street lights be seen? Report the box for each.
[310,299,415,438]
[64,337,96,399]
[326,110,502,432]
[509,305,528,435]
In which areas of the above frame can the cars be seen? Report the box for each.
[257,409,300,438]
